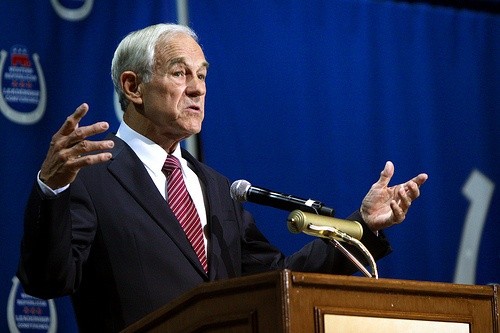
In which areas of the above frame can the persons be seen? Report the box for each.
[15,22,429,333]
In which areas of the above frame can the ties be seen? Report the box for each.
[160,154,209,275]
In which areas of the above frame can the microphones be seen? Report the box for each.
[229,179,335,217]
